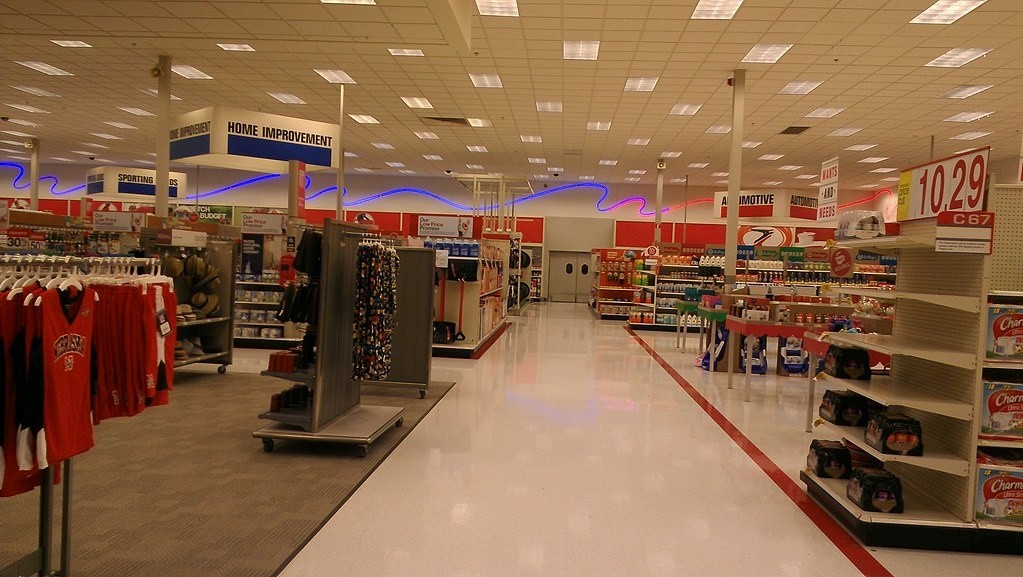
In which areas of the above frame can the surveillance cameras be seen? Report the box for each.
[89,157,94,160]
[1,117,9,121]
[446,171,451,173]
[554,174,559,176]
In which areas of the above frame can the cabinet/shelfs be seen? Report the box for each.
[174,316,235,375]
[588,270,604,319]
[479,257,504,297]
[656,265,897,328]
[234,282,303,341]
[626,269,657,327]
[598,288,640,315]
[529,269,543,298]
[799,169,997,551]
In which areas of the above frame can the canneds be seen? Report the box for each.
[671,271,698,280]
[758,271,867,285]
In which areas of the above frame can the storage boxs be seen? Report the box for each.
[741,309,770,322]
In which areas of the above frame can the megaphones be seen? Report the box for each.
[150,65,163,79]
[657,162,667,169]
[25,141,36,148]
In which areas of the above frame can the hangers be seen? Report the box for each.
[0,253,174,306]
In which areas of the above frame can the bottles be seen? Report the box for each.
[407,235,478,257]
[665,248,725,267]
[628,310,653,323]
[656,313,706,326]
[880,255,897,273]
[765,285,774,300]
[790,285,797,296]
[758,271,867,284]
[670,271,698,279]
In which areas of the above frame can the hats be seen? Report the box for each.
[162,252,223,321]
[175,337,205,360]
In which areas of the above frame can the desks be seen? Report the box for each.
[725,314,829,402]
[800,328,891,433]
[697,305,729,371]
[675,299,701,352]
